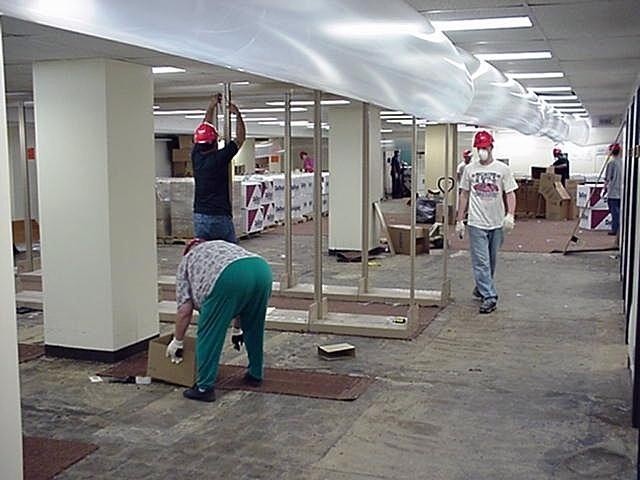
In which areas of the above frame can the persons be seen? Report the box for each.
[166,237,272,402]
[552,149,569,187]
[391,151,402,198]
[455,132,519,314]
[456,149,472,195]
[603,143,622,236]
[299,152,313,172]
[191,94,246,244]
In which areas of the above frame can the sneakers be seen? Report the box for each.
[474,285,499,312]
[244,373,261,385]
[182,385,213,402]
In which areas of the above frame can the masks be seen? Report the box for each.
[478,149,489,161]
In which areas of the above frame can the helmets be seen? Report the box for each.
[473,130,495,149]
[193,121,219,146]
[463,149,473,157]
[554,148,562,156]
[183,236,206,257]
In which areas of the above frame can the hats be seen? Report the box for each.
[607,142,622,151]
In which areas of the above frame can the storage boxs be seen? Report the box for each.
[387,224,429,256]
[150,134,329,240]
[504,163,614,234]
[145,331,198,389]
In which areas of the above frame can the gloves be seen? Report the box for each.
[456,220,467,238]
[230,325,245,350]
[165,338,184,365]
[501,214,516,231]
